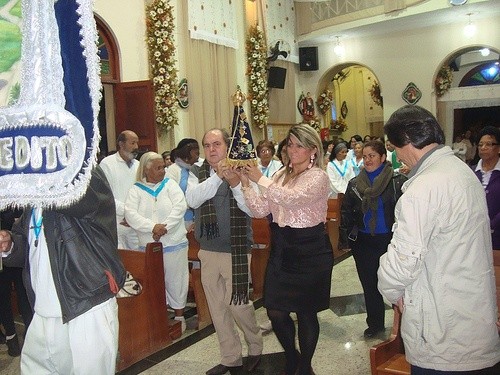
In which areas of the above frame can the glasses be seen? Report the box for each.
[260,149,272,154]
[479,142,498,148]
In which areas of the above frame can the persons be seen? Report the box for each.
[254,137,288,178]
[453,127,477,166]
[0,206,33,357]
[98,130,141,249]
[158,138,205,231]
[470,124,500,266]
[230,124,334,375]
[0,162,126,375]
[377,105,499,375]
[338,140,409,336]
[124,152,189,333]
[185,127,264,375]
[320,135,412,194]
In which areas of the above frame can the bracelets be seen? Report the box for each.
[241,183,252,193]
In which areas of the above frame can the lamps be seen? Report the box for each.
[333,35,345,58]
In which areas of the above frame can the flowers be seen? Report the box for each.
[231,23,270,160]
[317,66,454,134]
[144,0,180,137]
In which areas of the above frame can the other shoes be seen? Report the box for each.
[206,365,241,375]
[7,334,22,357]
[243,356,261,372]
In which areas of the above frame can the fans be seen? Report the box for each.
[331,68,352,84]
[267,38,291,64]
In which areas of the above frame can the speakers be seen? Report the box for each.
[299,47,319,71]
[268,67,286,89]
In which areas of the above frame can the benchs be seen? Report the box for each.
[116,192,500,375]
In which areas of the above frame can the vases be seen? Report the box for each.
[330,129,342,136]
